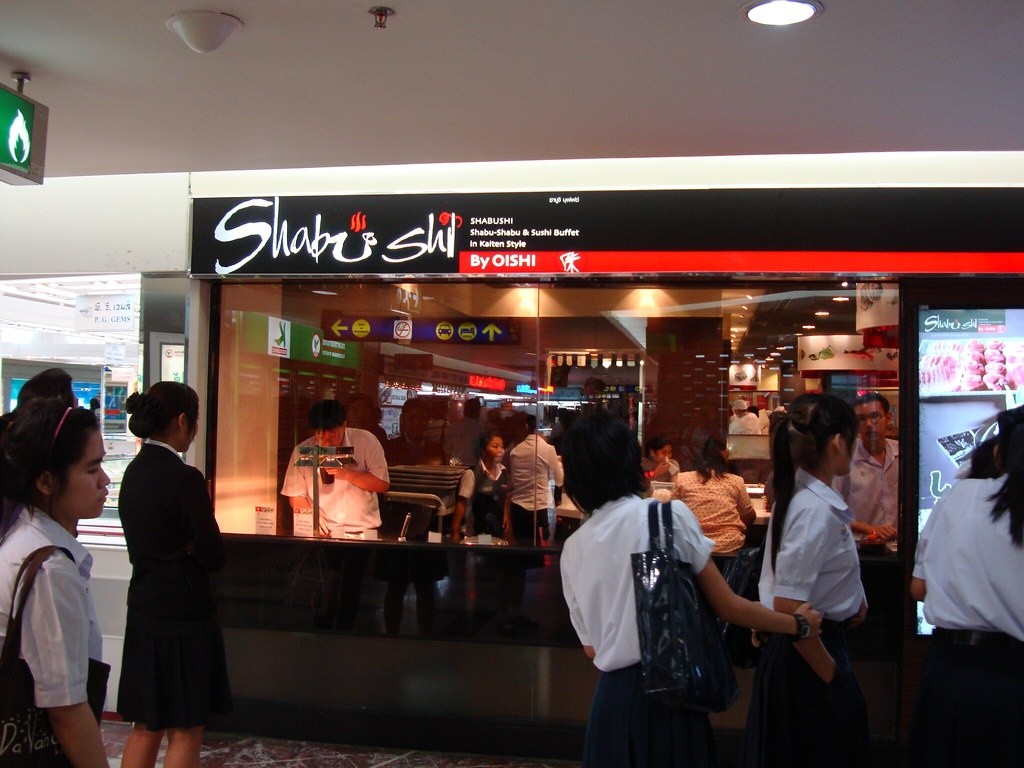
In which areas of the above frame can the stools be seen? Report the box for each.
[714,551,738,578]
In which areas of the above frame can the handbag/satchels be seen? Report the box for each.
[630,500,739,716]
[0,545,112,768]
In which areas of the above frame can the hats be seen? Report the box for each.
[733,400,747,410]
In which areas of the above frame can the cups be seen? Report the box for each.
[320,467,335,485]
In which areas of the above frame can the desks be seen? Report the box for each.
[557,483,772,525]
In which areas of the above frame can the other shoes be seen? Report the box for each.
[495,622,520,638]
[512,614,538,629]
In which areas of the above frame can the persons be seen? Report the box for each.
[451,431,512,543]
[670,435,756,569]
[343,392,531,475]
[510,413,564,544]
[729,399,770,434]
[641,435,680,483]
[904,407,1023,768]
[118,380,232,768]
[280,399,391,539]
[734,391,877,768]
[835,392,898,549]
[0,364,112,768]
[560,407,824,768]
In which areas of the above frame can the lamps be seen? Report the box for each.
[293,446,358,469]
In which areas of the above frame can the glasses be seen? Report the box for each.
[859,414,886,423]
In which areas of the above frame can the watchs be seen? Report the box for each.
[794,613,811,641]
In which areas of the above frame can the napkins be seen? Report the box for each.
[665,457,678,476]
